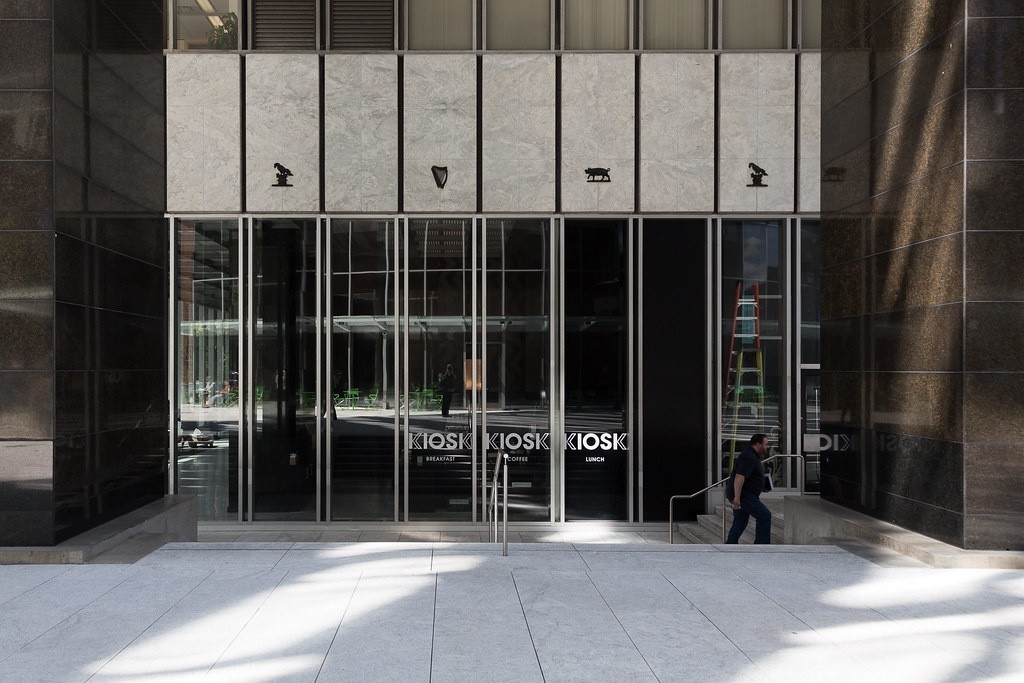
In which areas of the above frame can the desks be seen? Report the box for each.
[343,390,363,411]
[409,389,430,412]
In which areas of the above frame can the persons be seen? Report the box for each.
[726,433,772,544]
[204,376,230,406]
[437,365,455,418]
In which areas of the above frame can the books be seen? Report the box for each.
[763,473,771,492]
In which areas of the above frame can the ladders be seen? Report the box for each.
[722,278,768,485]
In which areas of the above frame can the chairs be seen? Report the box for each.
[193,381,446,414]
